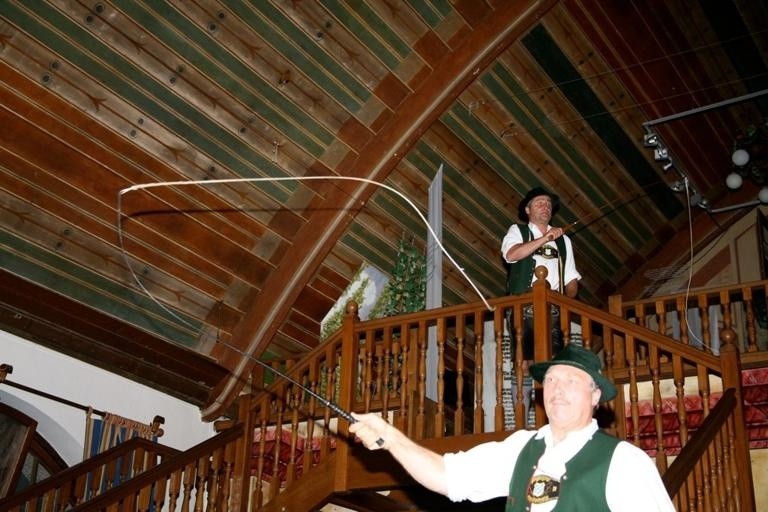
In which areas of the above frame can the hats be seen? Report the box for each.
[529,344,618,402]
[517,186,559,223]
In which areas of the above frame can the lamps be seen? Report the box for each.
[726,124,767,205]
[643,131,711,209]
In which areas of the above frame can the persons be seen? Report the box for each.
[347,342,676,512]
[500,187,582,431]
